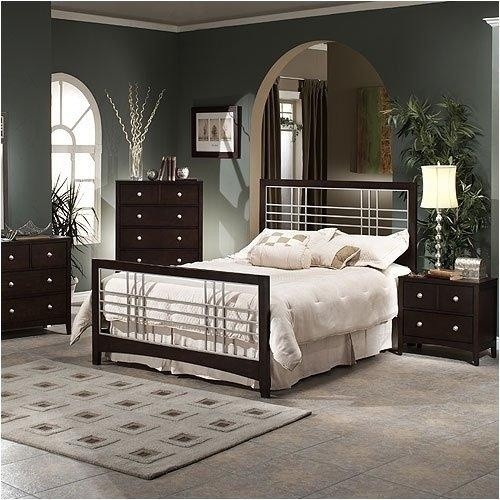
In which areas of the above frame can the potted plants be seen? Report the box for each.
[52,174,99,299]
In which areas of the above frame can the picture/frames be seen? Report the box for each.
[191,105,239,159]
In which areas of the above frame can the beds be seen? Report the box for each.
[92,180,418,399]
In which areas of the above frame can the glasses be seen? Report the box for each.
[408,271,426,279]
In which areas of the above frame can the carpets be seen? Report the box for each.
[2,356,311,479]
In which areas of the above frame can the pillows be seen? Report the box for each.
[229,228,410,271]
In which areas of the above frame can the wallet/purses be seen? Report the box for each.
[430,269,460,279]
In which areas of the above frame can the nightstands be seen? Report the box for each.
[398,274,499,366]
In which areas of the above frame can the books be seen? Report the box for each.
[157,155,177,182]
[431,269,463,281]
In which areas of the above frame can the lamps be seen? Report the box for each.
[420,161,459,280]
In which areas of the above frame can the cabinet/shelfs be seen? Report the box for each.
[2,234,71,334]
[115,179,203,266]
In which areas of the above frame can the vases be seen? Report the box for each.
[128,147,143,181]
